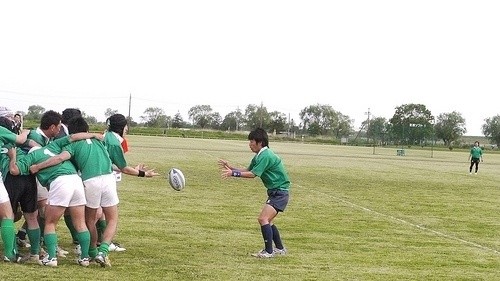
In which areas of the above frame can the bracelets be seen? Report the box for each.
[231,171,241,177]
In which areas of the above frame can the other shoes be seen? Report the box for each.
[107,243,127,252]
[256,250,275,258]
[94,255,111,268]
[76,257,89,267]
[2,237,69,267]
[273,247,286,255]
[74,244,81,255]
[475,173,478,175]
[469,172,472,174]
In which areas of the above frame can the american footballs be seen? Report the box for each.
[168,168,185,191]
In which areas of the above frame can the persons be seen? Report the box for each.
[0,108,160,267]
[468,141,483,174]
[217,128,290,257]
